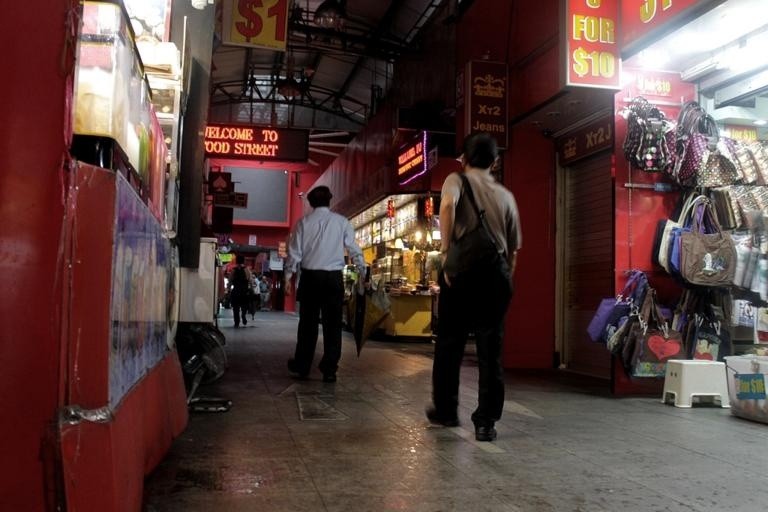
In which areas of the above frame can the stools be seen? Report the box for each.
[186,325,230,410]
[661,356,730,411]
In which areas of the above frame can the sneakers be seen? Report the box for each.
[424,406,459,426]
[323,374,336,382]
[286,357,312,380]
[474,425,500,441]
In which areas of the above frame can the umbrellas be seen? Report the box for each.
[345,265,390,361]
[247,287,256,322]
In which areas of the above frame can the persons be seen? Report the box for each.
[227,253,255,330]
[250,272,260,297]
[426,136,520,441]
[259,275,269,310]
[284,185,371,381]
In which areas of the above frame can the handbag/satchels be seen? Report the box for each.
[443,219,511,294]
[723,354,767,421]
[623,96,739,189]
[652,191,737,288]
[586,269,731,382]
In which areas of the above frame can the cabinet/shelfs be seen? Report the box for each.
[73,0,187,511]
[707,134,768,356]
[338,193,438,340]
